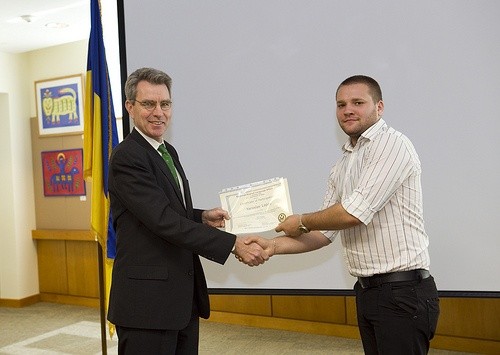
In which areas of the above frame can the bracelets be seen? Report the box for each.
[273,238,277,255]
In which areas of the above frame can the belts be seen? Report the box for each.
[359,270,429,285]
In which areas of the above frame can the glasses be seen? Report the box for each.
[133,98,172,110]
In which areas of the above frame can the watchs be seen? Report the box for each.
[298,214,311,234]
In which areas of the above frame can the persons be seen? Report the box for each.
[107,67,270,355]
[235,75,440,355]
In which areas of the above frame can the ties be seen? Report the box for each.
[158,143,180,189]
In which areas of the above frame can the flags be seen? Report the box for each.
[85,0,119,339]
